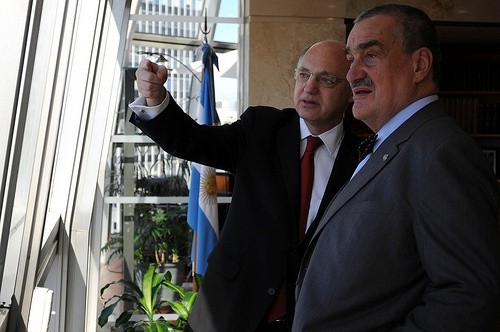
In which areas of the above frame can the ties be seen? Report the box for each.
[299,135,324,244]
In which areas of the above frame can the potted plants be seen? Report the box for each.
[101,201,197,309]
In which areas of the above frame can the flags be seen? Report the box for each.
[187,44,220,278]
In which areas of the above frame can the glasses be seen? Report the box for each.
[293,68,347,89]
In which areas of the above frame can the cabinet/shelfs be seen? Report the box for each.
[341,89,500,183]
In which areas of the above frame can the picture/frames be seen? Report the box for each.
[481,146,499,178]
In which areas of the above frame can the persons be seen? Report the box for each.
[129,40,371,332]
[291,4,500,332]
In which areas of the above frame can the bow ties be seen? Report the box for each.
[358,134,378,163]
